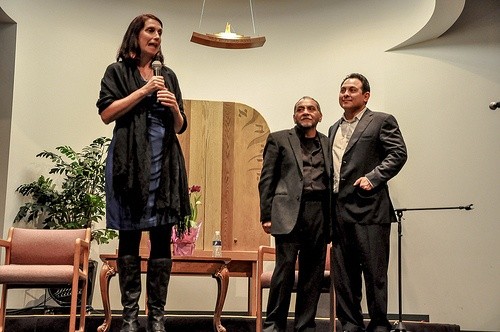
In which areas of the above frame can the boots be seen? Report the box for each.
[145,259,172,332]
[116,256,142,332]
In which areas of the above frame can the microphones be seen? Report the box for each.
[152,60,163,104]
[488,101,500,110]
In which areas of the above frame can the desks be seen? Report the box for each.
[97,254,232,332]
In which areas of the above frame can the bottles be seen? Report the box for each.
[212,231,222,257]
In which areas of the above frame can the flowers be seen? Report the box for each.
[179,185,205,227]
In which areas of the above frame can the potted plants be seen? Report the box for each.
[14,136,120,315]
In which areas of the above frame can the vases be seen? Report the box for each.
[172,222,202,256]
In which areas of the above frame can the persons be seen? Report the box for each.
[327,73,408,332]
[95,16,193,332]
[257,95,331,332]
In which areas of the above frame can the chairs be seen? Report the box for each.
[255,242,337,332]
[0,226,90,332]
